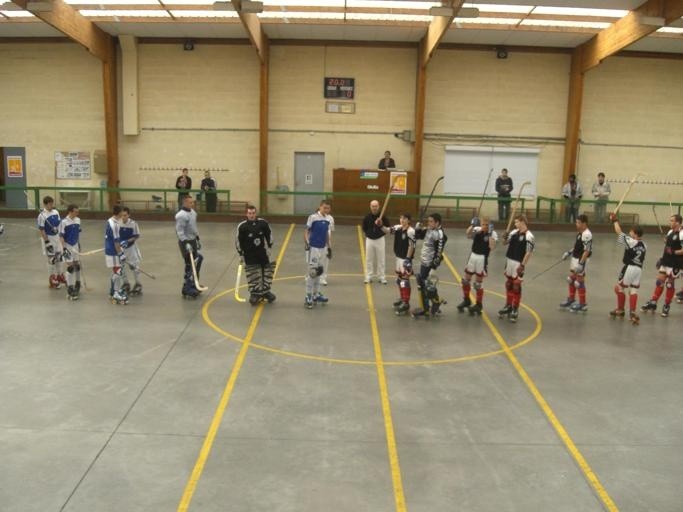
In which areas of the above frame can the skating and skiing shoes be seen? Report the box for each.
[558,297,576,310]
[498,304,512,317]
[411,306,429,318]
[57,273,67,284]
[675,289,683,303]
[628,311,640,325]
[120,283,131,293]
[469,302,483,314]
[609,310,625,320]
[509,309,519,322]
[249,293,264,305]
[570,304,588,314]
[128,285,143,296]
[263,292,276,303]
[312,292,329,304]
[111,291,128,305]
[49,273,61,289]
[392,297,403,307]
[661,305,670,319]
[431,297,447,315]
[65,286,79,299]
[640,301,657,314]
[304,294,314,308]
[457,297,472,312]
[182,282,199,300]
[393,301,410,314]
[74,285,81,293]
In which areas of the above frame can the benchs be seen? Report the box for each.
[194,202,248,213]
[421,207,477,219]
[585,212,638,225]
[511,209,554,223]
[119,200,173,212]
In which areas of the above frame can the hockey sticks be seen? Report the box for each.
[123,260,157,280]
[23,190,54,229]
[505,181,532,235]
[235,262,246,302]
[71,247,106,256]
[522,259,565,284]
[78,243,95,291]
[379,174,407,220]
[652,203,665,240]
[612,172,645,215]
[668,191,675,217]
[477,168,495,218]
[419,177,444,223]
[190,251,208,291]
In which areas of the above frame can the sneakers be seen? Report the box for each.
[364,277,373,284]
[378,278,388,284]
[319,278,328,286]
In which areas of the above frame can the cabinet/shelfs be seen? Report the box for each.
[58,188,93,212]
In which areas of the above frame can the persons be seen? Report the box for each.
[102,203,132,301]
[377,149,395,169]
[119,207,143,297]
[494,168,515,225]
[413,213,448,315]
[58,205,85,300]
[609,211,649,322]
[301,201,330,309]
[638,214,683,315]
[173,167,192,211]
[36,194,68,290]
[373,211,418,313]
[175,194,204,296]
[561,172,585,229]
[313,207,335,289]
[458,217,501,312]
[498,214,538,319]
[558,215,595,310]
[197,170,219,212]
[674,226,683,304]
[233,204,276,305]
[359,199,392,286]
[589,172,612,225]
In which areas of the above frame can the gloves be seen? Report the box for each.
[45,240,54,253]
[239,256,244,265]
[416,222,423,229]
[119,240,129,249]
[666,247,675,255]
[471,217,479,227]
[656,258,662,270]
[186,243,193,253]
[266,248,273,257]
[517,265,525,278]
[403,257,413,276]
[562,251,571,260]
[326,247,332,259]
[118,252,127,262]
[196,239,202,250]
[502,230,509,240]
[63,248,72,260]
[575,264,583,273]
[429,269,438,279]
[488,223,494,234]
[610,213,617,222]
[304,242,310,252]
